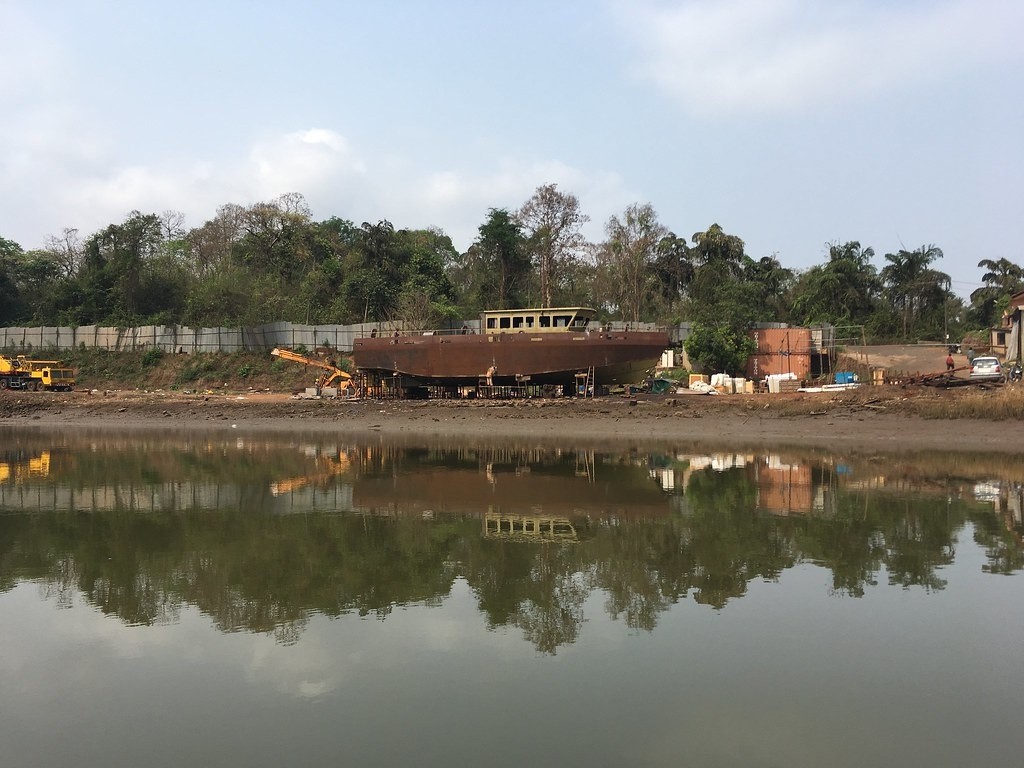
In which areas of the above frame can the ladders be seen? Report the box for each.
[584,450,594,481]
[584,366,595,398]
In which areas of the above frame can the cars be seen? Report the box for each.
[970,357,1004,382]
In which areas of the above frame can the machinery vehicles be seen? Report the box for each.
[271,348,372,399]
[0,355,76,392]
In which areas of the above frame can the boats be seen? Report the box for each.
[354,307,668,385]
[355,468,672,544]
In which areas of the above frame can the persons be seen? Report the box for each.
[487,367,495,386]
[966,347,974,363]
[946,354,954,370]
[394,328,399,344]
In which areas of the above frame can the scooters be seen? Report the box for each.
[1009,361,1023,382]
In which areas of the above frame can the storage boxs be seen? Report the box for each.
[689,374,754,394]
